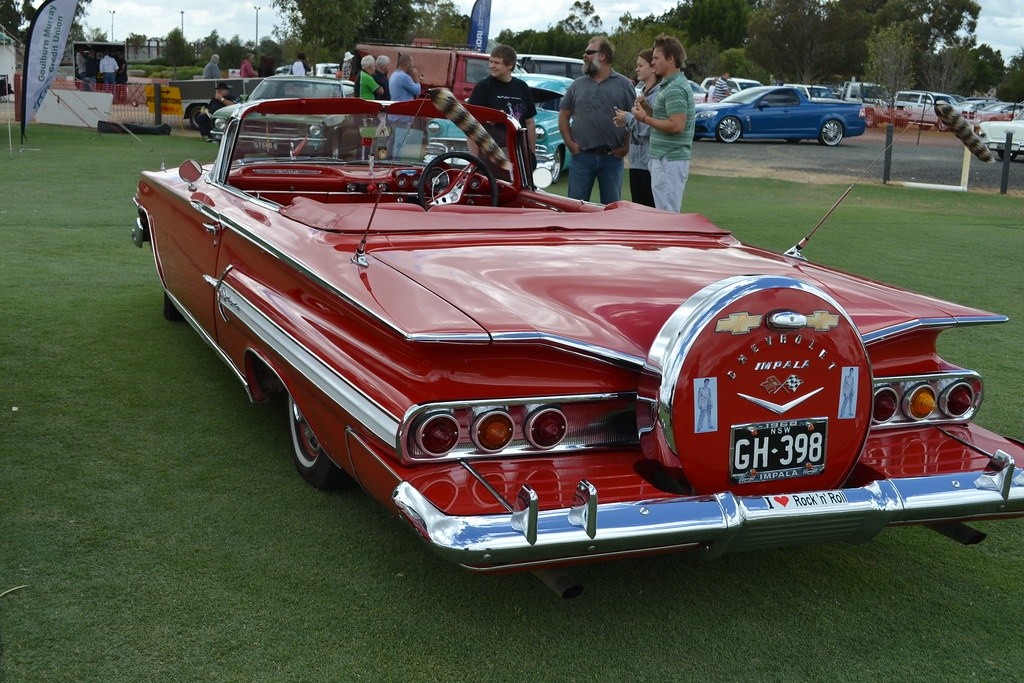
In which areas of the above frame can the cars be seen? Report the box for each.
[270,65,294,79]
[210,74,358,158]
[700,77,763,103]
[305,61,343,85]
[633,79,709,109]
[425,73,584,186]
[783,83,848,104]
[892,86,1024,163]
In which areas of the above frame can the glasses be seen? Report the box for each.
[584,50,601,55]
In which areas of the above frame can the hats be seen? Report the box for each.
[214,83,232,89]
[343,52,354,61]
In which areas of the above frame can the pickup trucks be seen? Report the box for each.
[693,86,866,149]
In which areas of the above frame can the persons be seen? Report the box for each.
[337,52,358,82]
[202,54,223,79]
[558,36,635,204]
[613,49,661,207]
[708,71,731,102]
[197,82,235,142]
[632,34,695,213]
[385,54,421,158]
[372,55,391,101]
[258,55,275,77]
[76,48,128,93]
[290,52,310,76]
[465,43,536,183]
[240,53,257,78]
[353,55,385,160]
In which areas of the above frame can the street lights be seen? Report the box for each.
[181,10,185,39]
[109,9,117,42]
[252,5,262,51]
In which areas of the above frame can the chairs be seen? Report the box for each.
[322,197,622,215]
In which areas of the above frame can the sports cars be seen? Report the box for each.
[129,93,1024,603]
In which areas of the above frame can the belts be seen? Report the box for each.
[585,147,612,154]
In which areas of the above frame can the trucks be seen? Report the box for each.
[352,33,532,104]
[831,81,910,129]
[70,38,128,86]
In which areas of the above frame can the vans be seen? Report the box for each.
[515,54,586,83]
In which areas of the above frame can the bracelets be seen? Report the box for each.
[221,98,224,102]
